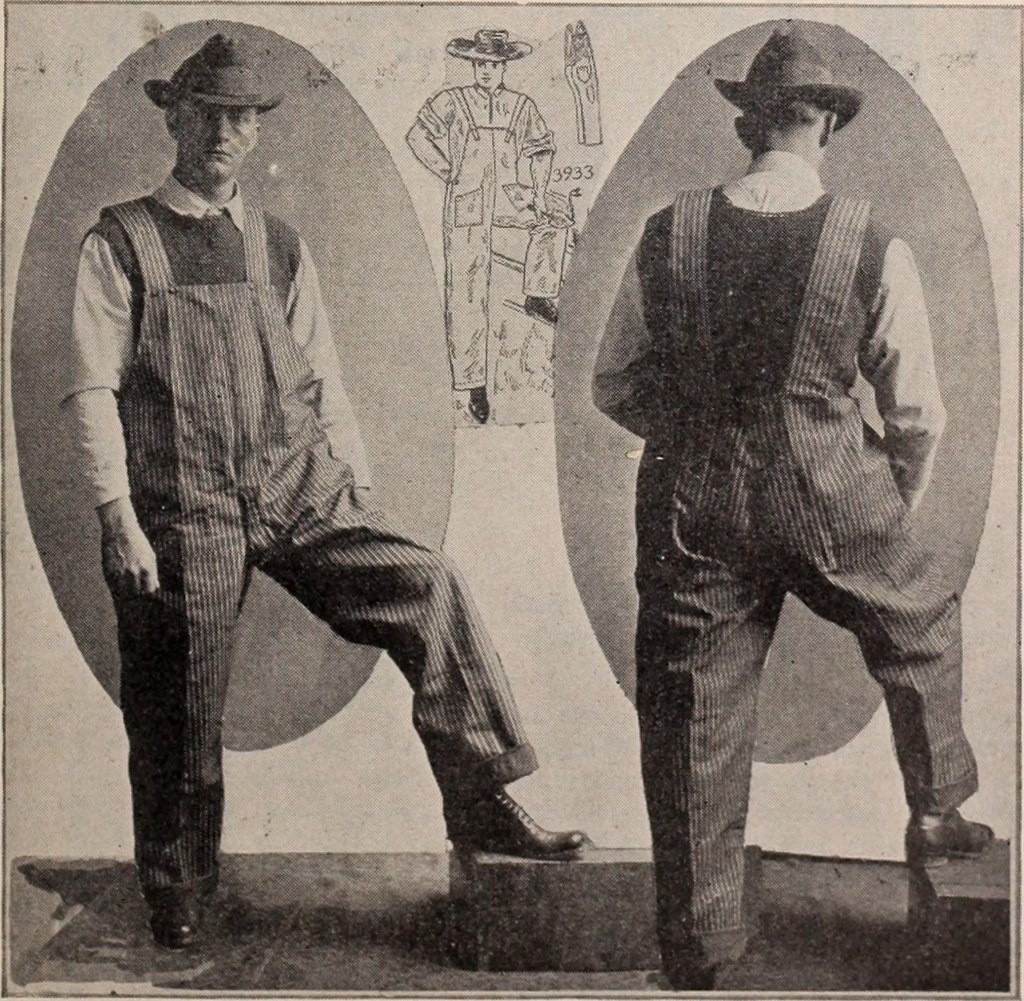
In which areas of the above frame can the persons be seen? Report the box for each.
[54,32,587,948]
[590,23,999,994]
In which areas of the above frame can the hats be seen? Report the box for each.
[714,27,865,130]
[142,34,283,113]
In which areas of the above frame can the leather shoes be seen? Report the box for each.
[445,789,588,863]
[905,805,995,871]
[151,898,209,953]
